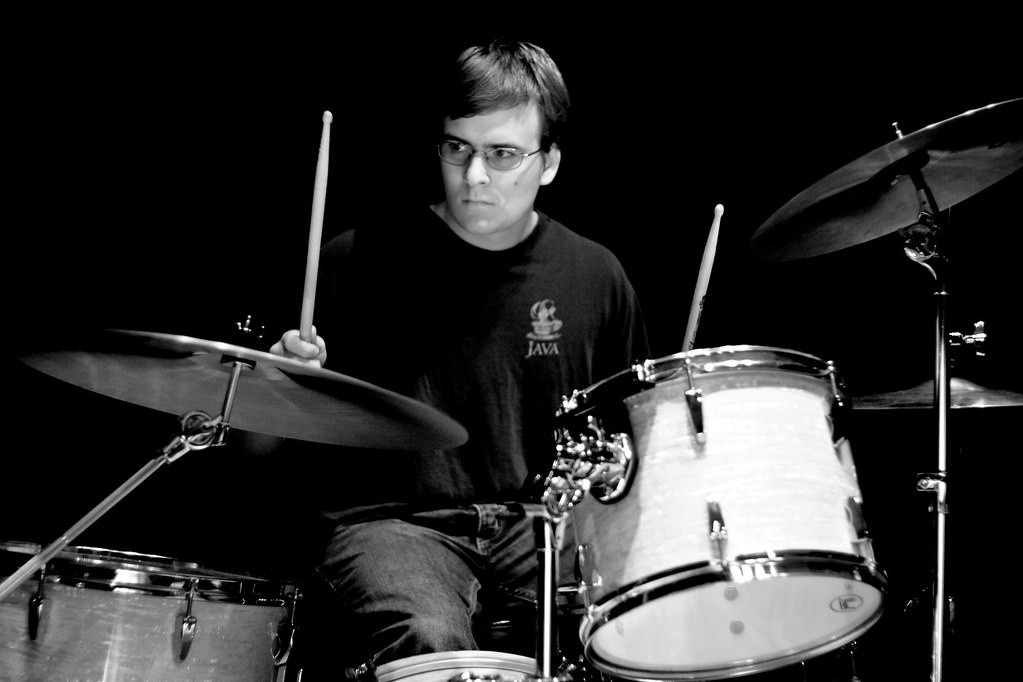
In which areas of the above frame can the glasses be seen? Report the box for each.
[437,131,543,171]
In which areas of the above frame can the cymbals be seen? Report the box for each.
[845,373,1023,415]
[4,312,473,458]
[747,95,1022,263]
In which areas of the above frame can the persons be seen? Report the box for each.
[244,39,651,682]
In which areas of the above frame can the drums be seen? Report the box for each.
[355,647,579,682]
[547,344,889,682]
[1,537,303,682]
